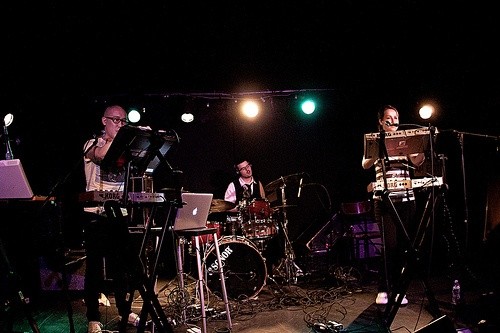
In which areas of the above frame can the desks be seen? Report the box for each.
[152,227,232,333]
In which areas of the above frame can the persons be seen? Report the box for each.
[83,104,140,333]
[362,104,425,306]
[224,159,266,204]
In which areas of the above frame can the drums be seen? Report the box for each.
[188,221,228,256]
[201,235,267,302]
[241,197,277,239]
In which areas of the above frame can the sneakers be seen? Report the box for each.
[88,321,103,333]
[394,293,409,305]
[375,291,388,304]
[118,312,148,326]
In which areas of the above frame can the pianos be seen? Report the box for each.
[362,125,454,326]
[77,123,185,333]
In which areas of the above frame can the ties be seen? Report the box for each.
[246,184,251,196]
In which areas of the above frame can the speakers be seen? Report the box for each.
[411,314,457,333]
[309,247,337,286]
[40,253,85,292]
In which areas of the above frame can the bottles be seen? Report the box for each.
[453,280,460,303]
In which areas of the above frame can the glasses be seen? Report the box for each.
[105,117,128,125]
[239,165,249,172]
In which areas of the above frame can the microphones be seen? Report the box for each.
[91,130,106,139]
[298,178,302,197]
[386,121,398,126]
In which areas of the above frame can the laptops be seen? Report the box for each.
[0,159,56,200]
[151,193,213,231]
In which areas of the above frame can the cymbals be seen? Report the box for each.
[158,187,190,192]
[263,170,302,192]
[208,199,236,213]
[273,204,298,208]
